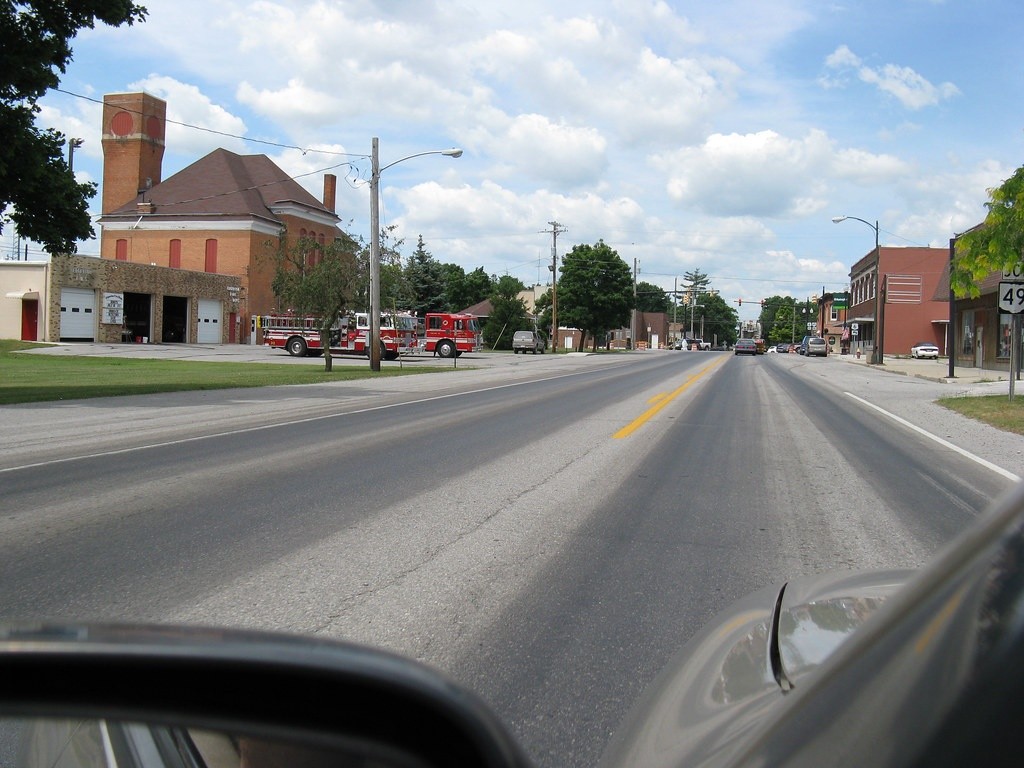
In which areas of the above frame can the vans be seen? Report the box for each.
[512,330,546,355]
[805,338,828,357]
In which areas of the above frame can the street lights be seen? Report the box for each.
[684,304,705,340]
[369,135,463,376]
[832,215,881,364]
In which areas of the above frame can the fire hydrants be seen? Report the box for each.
[857,348,861,359]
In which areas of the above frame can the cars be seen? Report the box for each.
[668,339,725,351]
[911,341,939,359]
[734,339,757,356]
[753,338,802,354]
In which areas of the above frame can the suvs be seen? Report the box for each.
[800,336,818,355]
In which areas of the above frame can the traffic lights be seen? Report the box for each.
[739,299,741,306]
[761,300,763,307]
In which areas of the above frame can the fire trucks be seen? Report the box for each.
[255,307,426,362]
[406,311,484,358]
